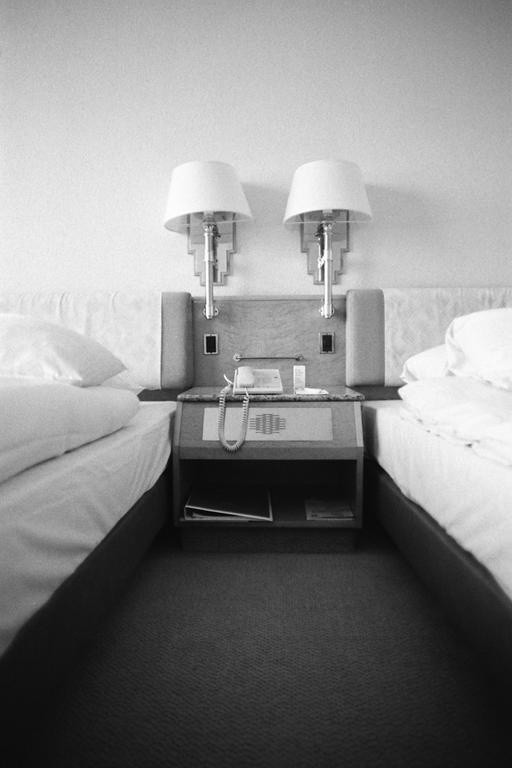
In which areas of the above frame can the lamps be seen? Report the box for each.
[166,159,253,321]
[283,158,372,315]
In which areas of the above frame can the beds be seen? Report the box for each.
[336,283,510,716]
[0,289,197,710]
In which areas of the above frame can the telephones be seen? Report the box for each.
[233,366,283,395]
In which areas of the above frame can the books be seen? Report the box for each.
[304,497,354,521]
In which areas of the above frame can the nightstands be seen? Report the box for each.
[174,385,367,552]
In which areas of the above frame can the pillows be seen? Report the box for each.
[400,344,463,384]
[0,313,129,391]
[448,310,510,394]
